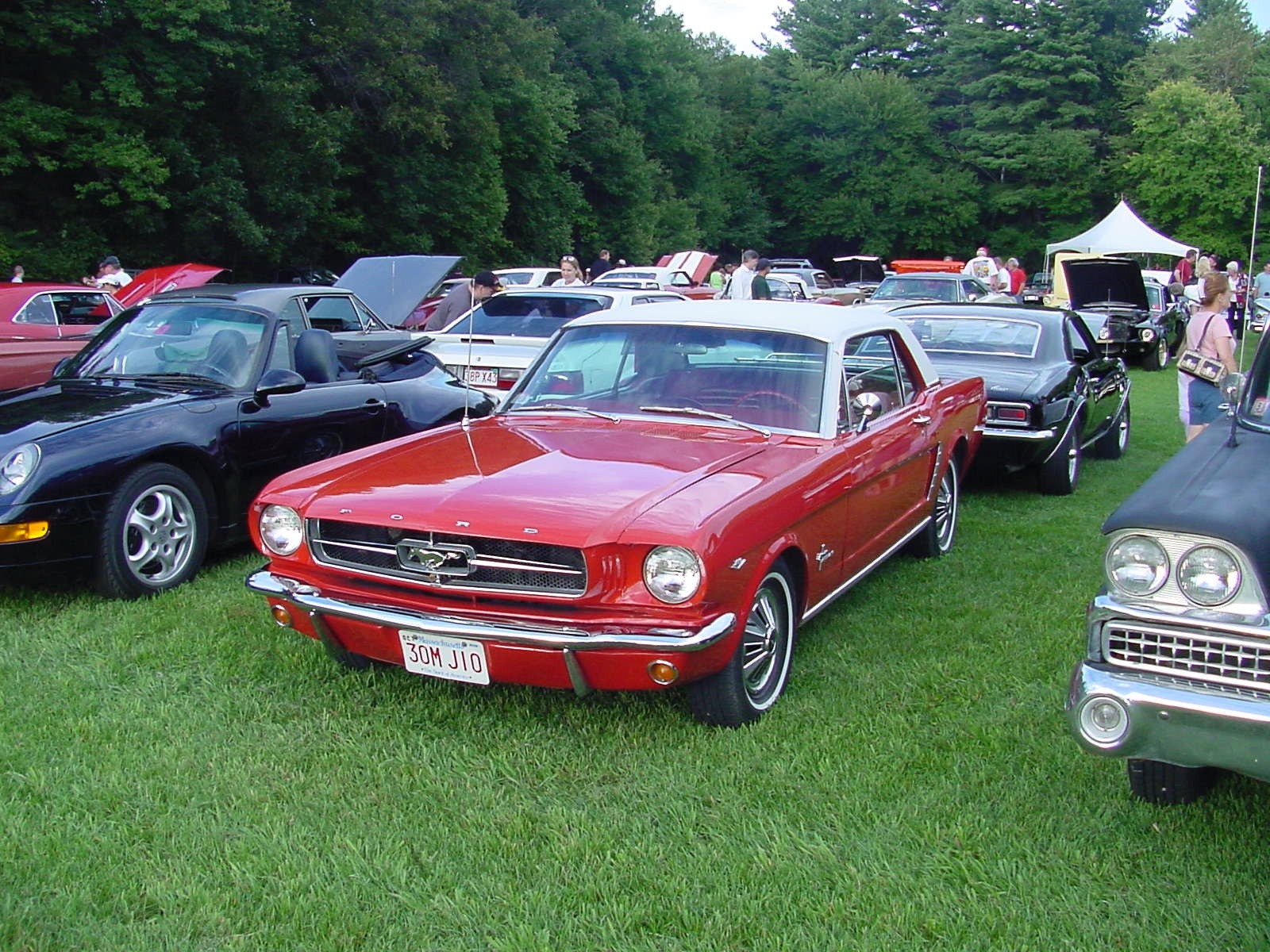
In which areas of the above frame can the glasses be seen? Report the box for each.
[562,256,577,262]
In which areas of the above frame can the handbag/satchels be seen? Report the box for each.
[1176,315,1226,385]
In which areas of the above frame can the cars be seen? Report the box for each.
[1062,314,1269,811]
[394,250,1268,500]
[0,252,503,604]
[0,262,236,394]
[240,299,991,731]
[839,302,1133,496]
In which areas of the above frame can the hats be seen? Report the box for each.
[474,271,503,288]
[100,255,118,268]
[755,258,774,268]
[977,247,988,252]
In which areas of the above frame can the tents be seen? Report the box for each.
[1042,192,1199,283]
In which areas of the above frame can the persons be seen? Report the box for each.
[83,256,133,296]
[963,247,1027,305]
[1145,250,1270,359]
[1177,272,1238,443]
[551,256,585,287]
[591,250,616,280]
[616,259,628,267]
[425,270,502,331]
[711,250,772,300]
[12,265,24,283]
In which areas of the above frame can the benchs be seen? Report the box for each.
[665,368,847,422]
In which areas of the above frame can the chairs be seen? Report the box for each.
[292,327,341,383]
[207,329,249,386]
[939,285,954,301]
[527,317,568,337]
[27,296,62,324]
[938,340,971,350]
